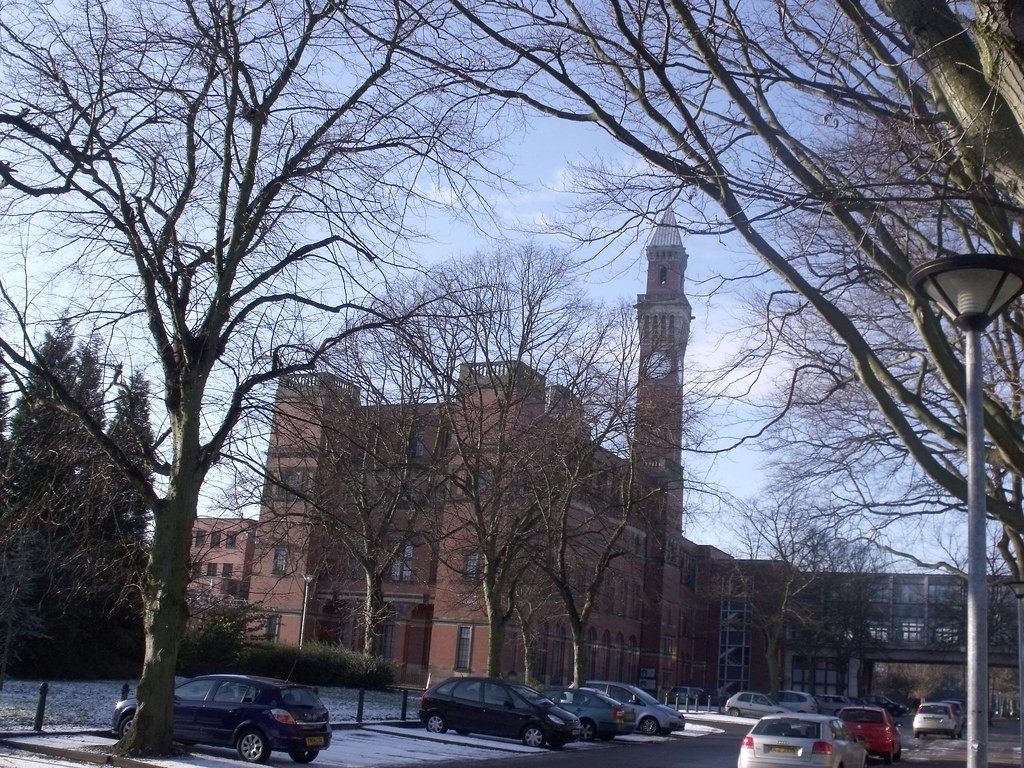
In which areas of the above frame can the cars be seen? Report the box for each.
[912,700,966,739]
[813,695,908,716]
[419,677,584,747]
[668,686,730,707]
[540,688,636,743]
[725,691,792,719]
[757,691,818,713]
[837,706,904,765]
[737,713,868,768]
[113,675,332,764]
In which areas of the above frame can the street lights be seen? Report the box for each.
[299,571,317,649]
[906,253,1024,768]
[1002,580,1024,768]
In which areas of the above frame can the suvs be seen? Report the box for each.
[560,680,686,736]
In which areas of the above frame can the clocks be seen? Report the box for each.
[643,351,671,380]
